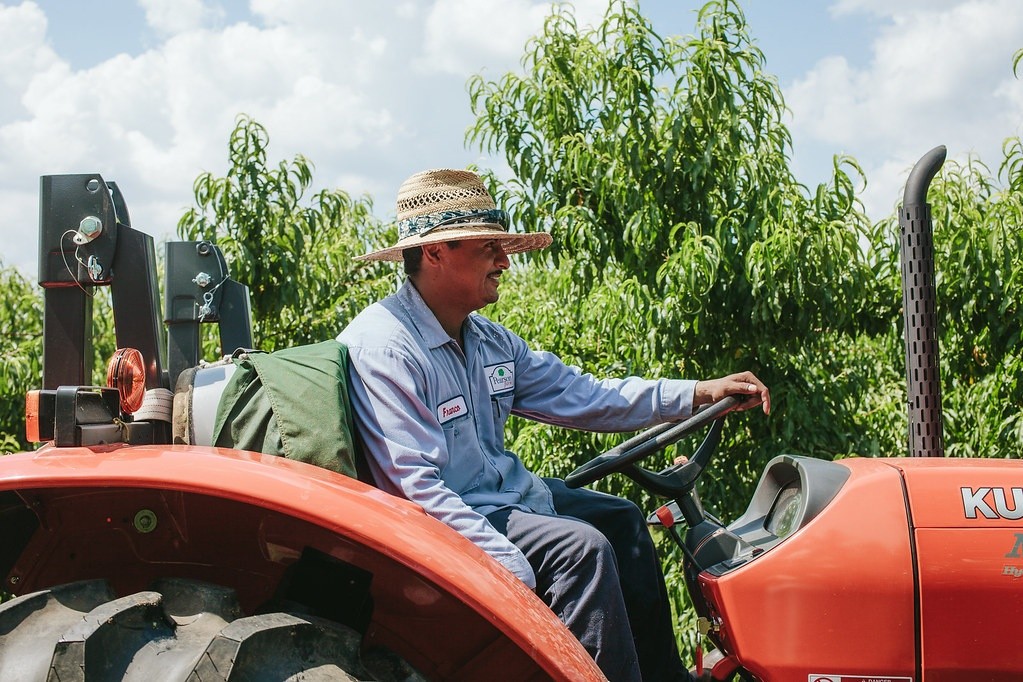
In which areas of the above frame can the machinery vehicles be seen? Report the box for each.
[2,167,1023,682]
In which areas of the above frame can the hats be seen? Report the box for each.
[350,169,553,262]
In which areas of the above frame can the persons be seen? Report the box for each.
[333,167,772,682]
